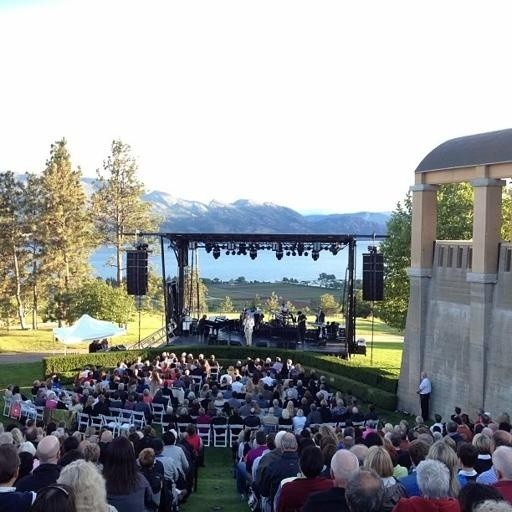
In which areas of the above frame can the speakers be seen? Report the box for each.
[277,342,297,349]
[230,340,240,345]
[362,253,383,300]
[218,340,228,345]
[127,253,148,294]
[256,342,267,347]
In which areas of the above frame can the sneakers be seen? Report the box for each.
[177,488,187,501]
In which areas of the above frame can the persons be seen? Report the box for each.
[0,417,203,511]
[232,405,512,511]
[415,370,435,423]
[192,303,325,348]
[88,337,110,353]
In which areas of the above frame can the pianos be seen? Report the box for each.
[204,319,221,336]
